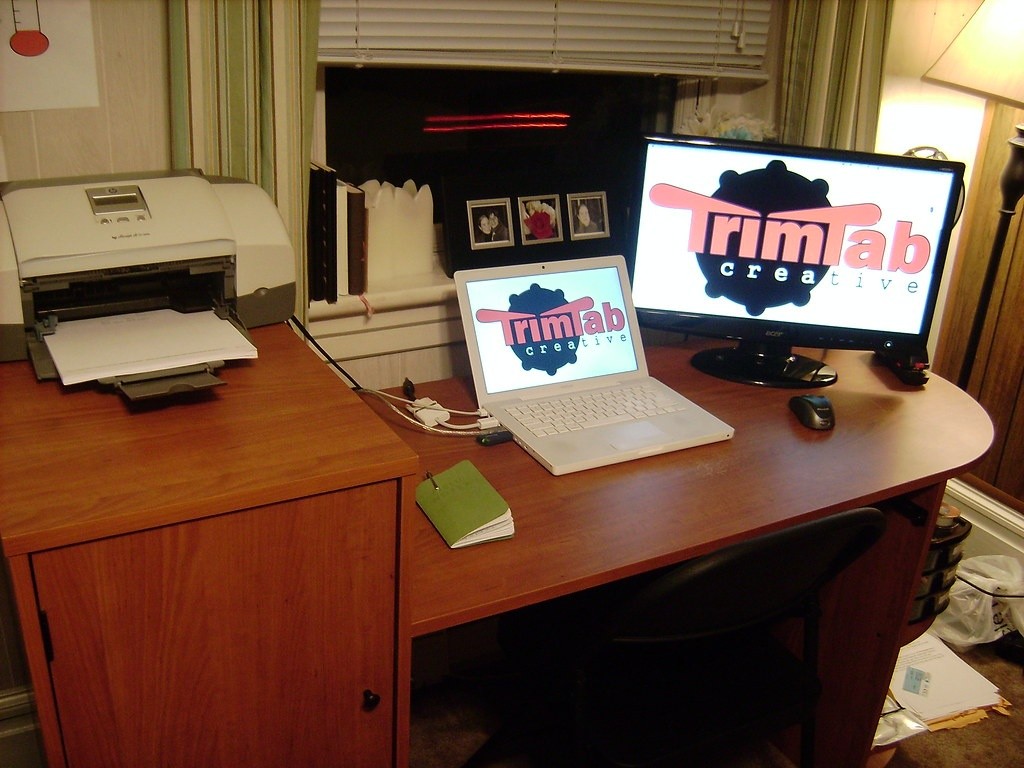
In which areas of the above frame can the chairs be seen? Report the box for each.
[494,505,887,768]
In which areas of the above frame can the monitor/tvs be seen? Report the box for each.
[626,132,966,389]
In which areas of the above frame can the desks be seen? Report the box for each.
[358,339,996,768]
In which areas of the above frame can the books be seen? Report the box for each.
[415,459,514,549]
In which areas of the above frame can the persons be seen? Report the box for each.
[575,204,598,234]
[478,210,509,243]
[524,202,555,238]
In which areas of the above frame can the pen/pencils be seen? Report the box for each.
[425,470,440,491]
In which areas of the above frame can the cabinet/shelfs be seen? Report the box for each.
[0,321,420,768]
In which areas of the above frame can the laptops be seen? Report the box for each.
[454,254,735,475]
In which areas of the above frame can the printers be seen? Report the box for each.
[0,167,297,403]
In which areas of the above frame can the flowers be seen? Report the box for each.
[677,105,778,142]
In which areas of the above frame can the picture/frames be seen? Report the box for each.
[466,198,515,250]
[518,193,564,246]
[566,191,611,241]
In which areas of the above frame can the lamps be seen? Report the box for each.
[920,0,1024,391]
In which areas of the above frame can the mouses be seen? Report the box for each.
[789,393,836,430]
